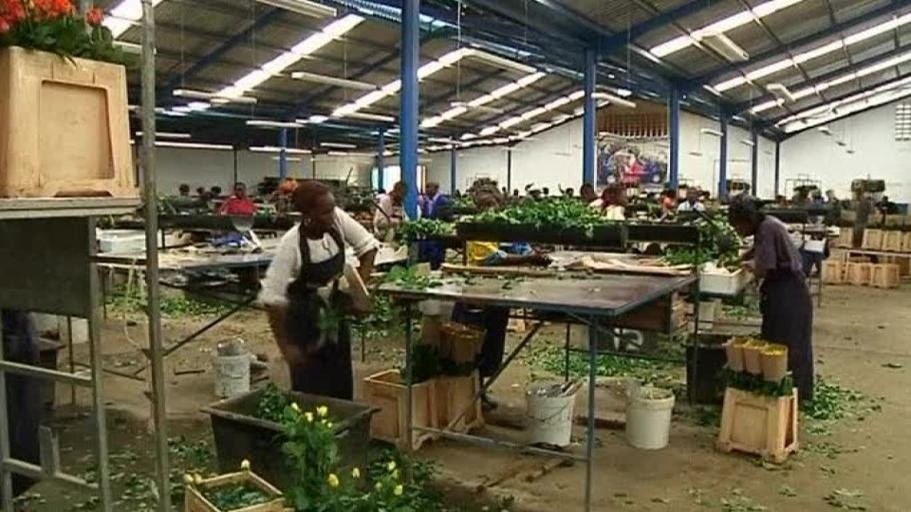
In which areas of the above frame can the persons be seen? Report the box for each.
[660,188,705,212]
[256,181,380,401]
[737,184,842,223]
[271,180,296,212]
[451,183,555,413]
[178,183,255,218]
[729,199,816,404]
[373,182,449,263]
[580,182,625,221]
[857,195,897,223]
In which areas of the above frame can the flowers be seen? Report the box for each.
[0,0,136,65]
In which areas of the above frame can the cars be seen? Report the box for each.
[597,150,667,186]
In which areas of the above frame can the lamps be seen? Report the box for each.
[102,0,910,162]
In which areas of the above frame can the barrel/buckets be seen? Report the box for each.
[209,351,252,399]
[522,382,577,449]
[623,388,676,450]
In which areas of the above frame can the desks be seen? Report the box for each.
[103,203,834,512]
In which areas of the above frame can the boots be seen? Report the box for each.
[479,376,498,412]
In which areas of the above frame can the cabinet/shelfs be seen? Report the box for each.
[0,0,171,512]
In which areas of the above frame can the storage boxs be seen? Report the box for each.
[822,227,911,290]
[0,45,139,196]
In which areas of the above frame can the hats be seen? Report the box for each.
[528,185,543,193]
